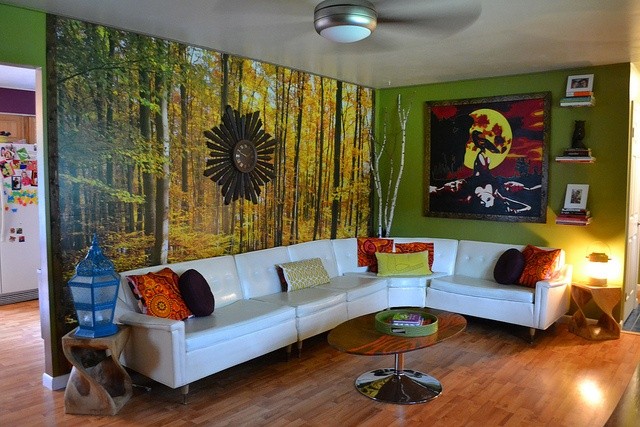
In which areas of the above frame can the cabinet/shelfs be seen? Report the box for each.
[556,101,595,227]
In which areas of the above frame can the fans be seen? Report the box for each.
[213,0,483,53]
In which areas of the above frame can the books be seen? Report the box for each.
[392,313,433,327]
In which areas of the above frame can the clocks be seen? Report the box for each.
[202,104,278,206]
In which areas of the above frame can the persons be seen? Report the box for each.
[1,147,5,156]
[21,172,32,185]
[13,178,19,187]
[575,190,580,198]
[572,81,578,88]
[581,79,587,87]
[6,151,13,159]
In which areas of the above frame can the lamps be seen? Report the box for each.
[313,5,378,43]
[586,240,613,287]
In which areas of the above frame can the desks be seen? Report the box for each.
[569,281,623,341]
[62,326,134,418]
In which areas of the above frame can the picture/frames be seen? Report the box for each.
[564,183,589,210]
[566,73,594,91]
[421,90,552,224]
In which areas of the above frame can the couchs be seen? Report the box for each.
[112,237,575,405]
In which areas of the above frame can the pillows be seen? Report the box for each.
[493,248,523,285]
[179,269,215,317]
[519,244,561,287]
[394,241,434,272]
[125,266,193,321]
[356,238,393,268]
[375,250,433,277]
[275,258,331,293]
